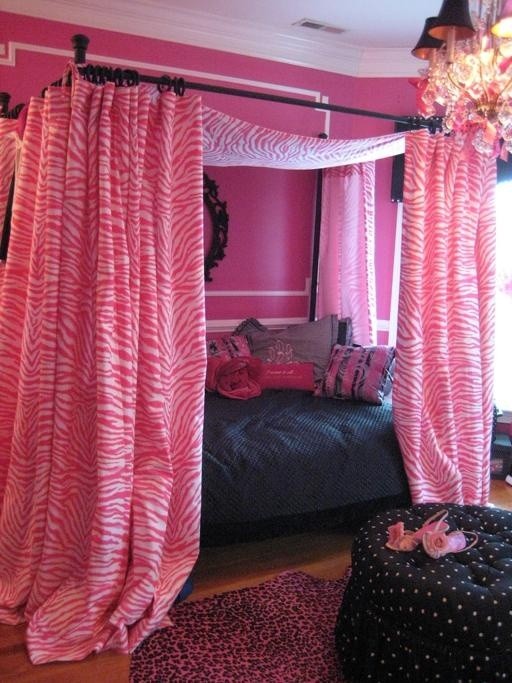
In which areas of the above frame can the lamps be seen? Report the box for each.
[410,3,512,165]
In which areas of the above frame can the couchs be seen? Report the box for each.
[336,502,511,682]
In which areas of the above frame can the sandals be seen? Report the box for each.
[422,530,479,559]
[385,509,449,552]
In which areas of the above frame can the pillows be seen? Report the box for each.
[312,342,397,406]
[231,314,339,392]
[353,342,395,397]
[337,316,353,345]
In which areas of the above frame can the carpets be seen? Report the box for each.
[127,567,349,682]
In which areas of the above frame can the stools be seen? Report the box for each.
[489,431,512,479]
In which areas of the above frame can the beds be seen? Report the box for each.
[0,35,500,558]
